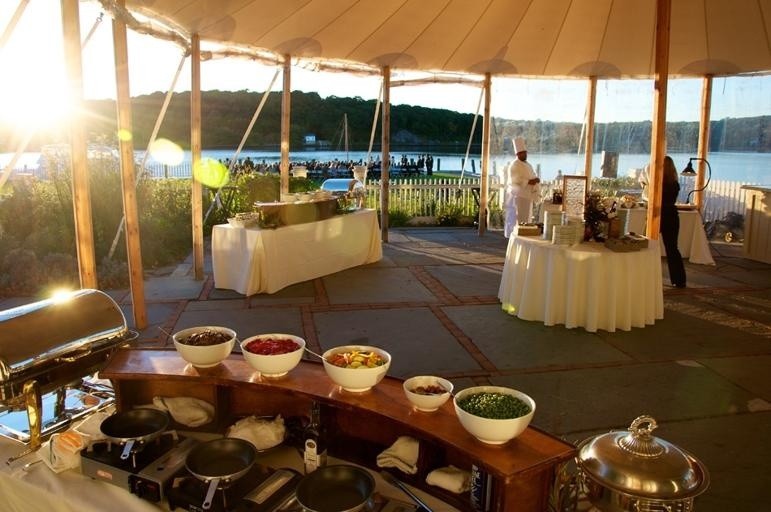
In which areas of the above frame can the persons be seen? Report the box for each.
[661,155,686,287]
[504,151,542,239]
[638,161,650,208]
[217,154,433,179]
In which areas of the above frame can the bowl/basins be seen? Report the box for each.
[239,333,305,378]
[321,345,391,393]
[403,375,453,413]
[170,326,237,368]
[453,385,536,446]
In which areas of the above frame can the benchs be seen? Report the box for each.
[288,163,425,178]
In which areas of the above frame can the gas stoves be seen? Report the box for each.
[78,433,200,503]
[281,490,420,512]
[169,463,299,512]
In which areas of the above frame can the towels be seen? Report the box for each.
[36,409,110,477]
[133,393,214,428]
[376,434,420,476]
[425,464,475,495]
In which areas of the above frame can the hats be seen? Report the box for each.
[513,137,527,154]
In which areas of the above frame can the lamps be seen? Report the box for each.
[674,157,712,211]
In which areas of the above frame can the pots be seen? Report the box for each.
[573,413,711,510]
[186,438,257,511]
[294,464,375,511]
[100,409,170,460]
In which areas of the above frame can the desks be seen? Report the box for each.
[469,187,500,231]
